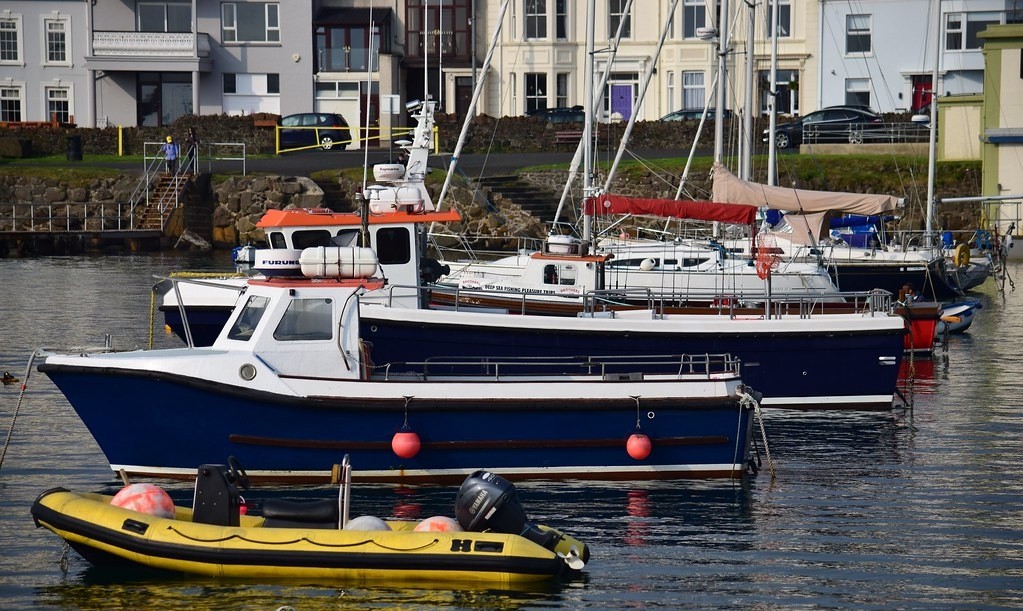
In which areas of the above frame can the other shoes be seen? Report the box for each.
[165,173,174,177]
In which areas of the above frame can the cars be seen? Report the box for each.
[277,112,352,151]
[762,104,885,149]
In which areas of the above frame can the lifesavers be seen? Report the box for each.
[954,243,970,268]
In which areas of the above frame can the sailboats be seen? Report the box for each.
[36,1,1015,493]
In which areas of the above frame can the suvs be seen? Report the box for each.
[525,104,587,125]
[659,107,739,122]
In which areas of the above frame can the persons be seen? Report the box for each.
[185,126,200,173]
[158,136,178,177]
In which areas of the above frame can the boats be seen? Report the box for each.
[31,455,594,593]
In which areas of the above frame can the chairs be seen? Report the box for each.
[332,453,349,529]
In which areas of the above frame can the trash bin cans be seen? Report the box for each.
[67,135,83,161]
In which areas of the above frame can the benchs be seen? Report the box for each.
[552,131,601,153]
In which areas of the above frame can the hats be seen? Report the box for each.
[165,136,172,144]
